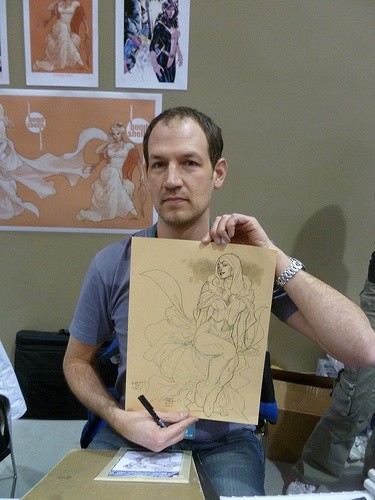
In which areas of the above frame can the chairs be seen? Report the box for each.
[16,331,77,421]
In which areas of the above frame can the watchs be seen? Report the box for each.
[275,256,307,291]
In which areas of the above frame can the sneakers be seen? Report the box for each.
[284,464,318,495]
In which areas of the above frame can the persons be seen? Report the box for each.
[63,106,375,500]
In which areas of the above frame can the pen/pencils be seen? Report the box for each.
[137,394,167,429]
